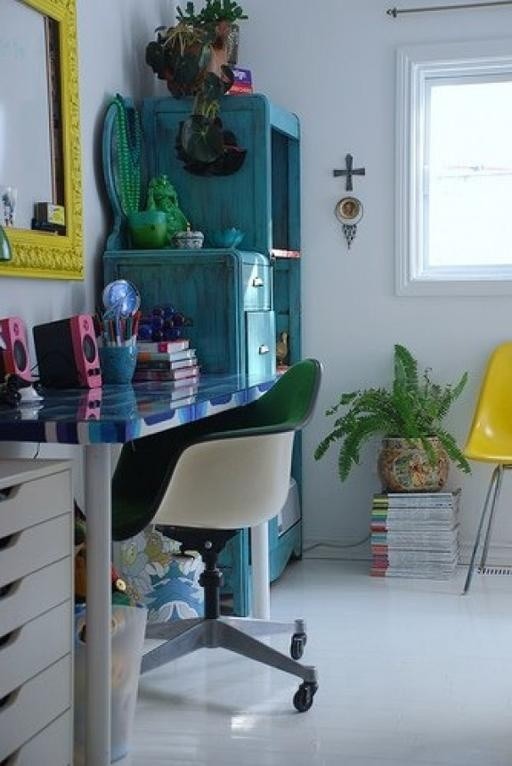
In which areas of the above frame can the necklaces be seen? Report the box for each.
[111,95,144,215]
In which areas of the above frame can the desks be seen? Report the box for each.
[1,375,282,766]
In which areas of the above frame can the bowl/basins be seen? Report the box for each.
[207,231,246,248]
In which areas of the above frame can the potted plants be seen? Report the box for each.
[145,0,249,98]
[314,345,471,492]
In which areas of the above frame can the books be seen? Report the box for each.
[132,338,202,381]
[223,63,254,97]
[370,488,465,579]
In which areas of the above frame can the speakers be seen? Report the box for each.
[34,314,102,389]
[39,388,103,421]
[0,318,32,389]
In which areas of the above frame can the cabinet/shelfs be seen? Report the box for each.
[0,458,75,765]
[140,94,302,585]
[102,251,276,617]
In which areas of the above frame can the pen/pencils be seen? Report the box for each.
[93,306,142,349]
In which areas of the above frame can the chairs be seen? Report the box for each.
[112,359,324,711]
[461,342,511,596]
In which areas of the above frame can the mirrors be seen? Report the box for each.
[102,97,139,250]
[0,0,83,279]
